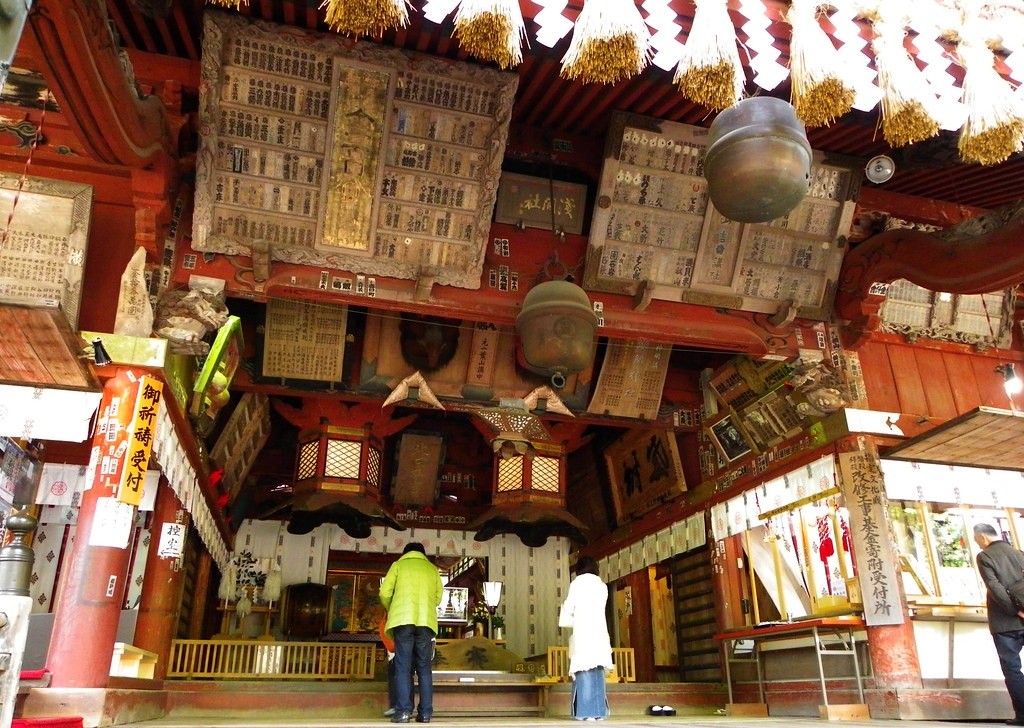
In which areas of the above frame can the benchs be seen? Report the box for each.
[109,642,158,680]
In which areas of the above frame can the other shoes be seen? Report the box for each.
[415,715,430,723]
[383,707,396,716]
[391,712,410,723]
[1006,718,1024,726]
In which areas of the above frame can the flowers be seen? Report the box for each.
[472,601,489,623]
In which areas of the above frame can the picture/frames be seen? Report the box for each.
[702,405,759,469]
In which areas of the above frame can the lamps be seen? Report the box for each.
[77,337,112,366]
[996,363,1022,394]
[482,581,503,615]
[865,154,895,184]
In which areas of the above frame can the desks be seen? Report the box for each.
[435,638,507,645]
[714,619,871,721]
[411,682,562,718]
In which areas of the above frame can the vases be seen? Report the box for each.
[475,622,484,636]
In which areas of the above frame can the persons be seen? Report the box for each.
[378,542,444,722]
[558,555,613,721]
[973,522,1024,726]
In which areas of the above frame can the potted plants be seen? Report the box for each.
[493,616,504,641]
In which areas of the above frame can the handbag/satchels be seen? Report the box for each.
[1007,580,1024,610]
